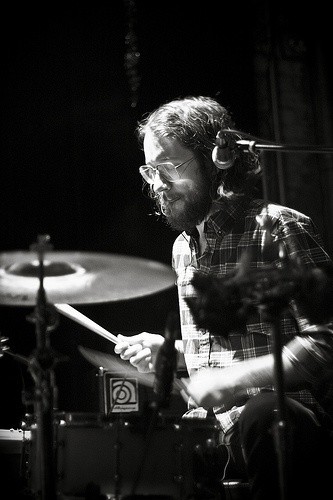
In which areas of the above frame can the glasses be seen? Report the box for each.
[138,156,195,185]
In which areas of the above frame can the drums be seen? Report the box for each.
[20,413,214,499]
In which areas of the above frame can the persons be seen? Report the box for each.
[113,95,333,500]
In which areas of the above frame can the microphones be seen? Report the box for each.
[212,130,236,170]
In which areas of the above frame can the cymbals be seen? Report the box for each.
[1,250,178,306]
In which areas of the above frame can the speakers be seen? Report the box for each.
[54,411,193,499]
